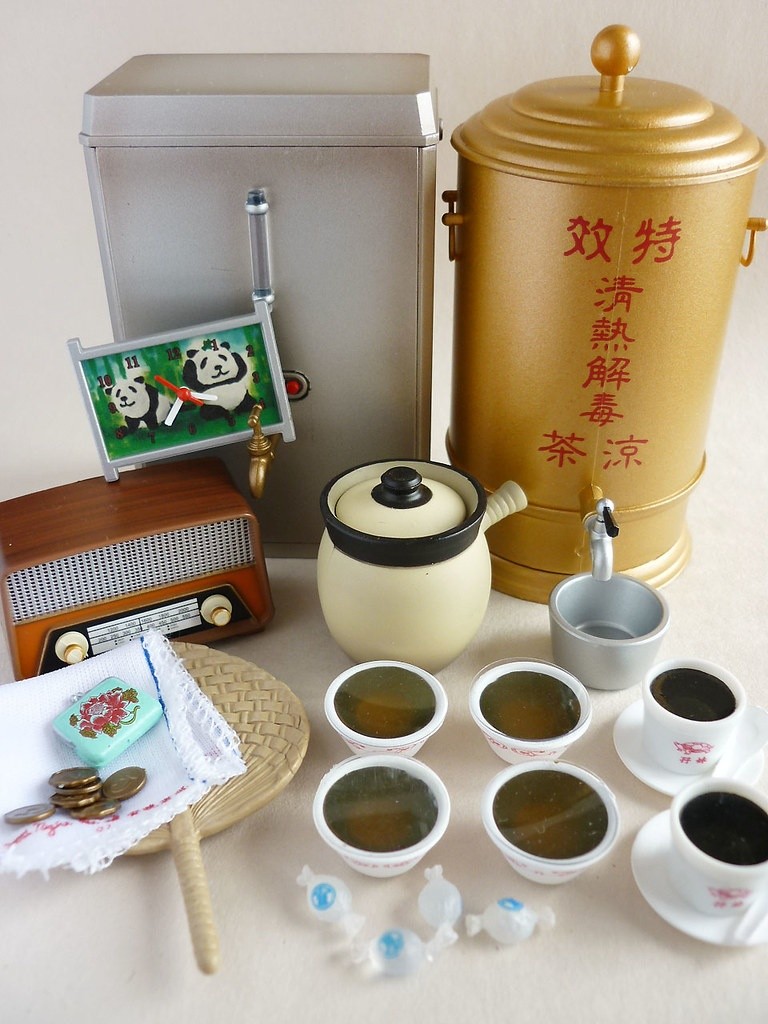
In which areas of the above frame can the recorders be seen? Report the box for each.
[1,455,276,682]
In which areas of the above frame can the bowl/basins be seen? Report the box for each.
[324,661,448,758]
[312,752,450,877]
[481,760,622,886]
[469,657,593,763]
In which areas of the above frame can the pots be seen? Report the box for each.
[316,456,529,675]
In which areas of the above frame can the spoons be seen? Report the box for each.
[713,706,768,777]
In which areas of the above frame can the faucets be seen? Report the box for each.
[245,400,283,501]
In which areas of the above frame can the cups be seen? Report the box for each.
[643,658,745,770]
[669,778,768,917]
[549,570,670,690]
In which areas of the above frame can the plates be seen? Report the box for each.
[613,700,768,795]
[631,808,768,947]
